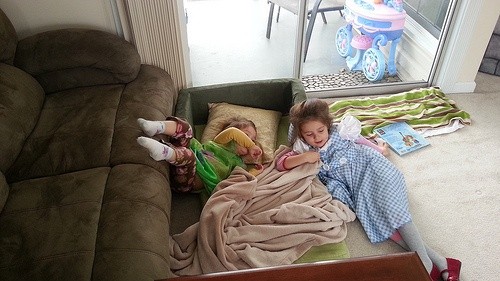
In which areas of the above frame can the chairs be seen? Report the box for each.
[266,0,346,63]
[169,77,308,194]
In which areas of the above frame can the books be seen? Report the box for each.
[374,121,430,156]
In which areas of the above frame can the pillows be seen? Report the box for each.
[200,102,282,163]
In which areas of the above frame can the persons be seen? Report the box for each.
[276,98,461,281]
[138,116,264,194]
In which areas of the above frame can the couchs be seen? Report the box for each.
[0,7,178,281]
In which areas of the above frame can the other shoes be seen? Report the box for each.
[430,263,441,281]
[441,258,461,281]
